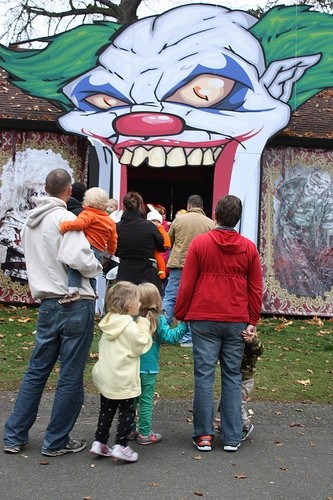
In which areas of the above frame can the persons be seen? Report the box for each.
[174,195,263,452]
[58,187,118,304]
[217,330,265,441]
[66,182,216,348]
[3,169,104,457]
[128,283,188,445]
[90,282,153,462]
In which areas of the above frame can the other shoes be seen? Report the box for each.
[192,434,212,450]
[242,424,254,441]
[224,443,241,450]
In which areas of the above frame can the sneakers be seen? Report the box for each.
[138,431,161,444]
[129,428,138,439]
[58,292,81,304]
[42,437,86,456]
[90,441,112,456]
[4,442,27,453]
[111,445,139,461]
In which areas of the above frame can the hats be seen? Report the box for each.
[147,211,162,224]
